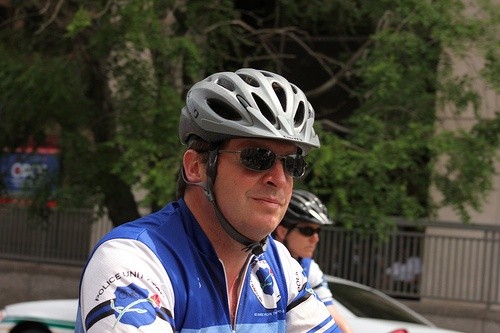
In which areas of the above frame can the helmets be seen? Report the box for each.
[178,68,322,148]
[282,188,334,225]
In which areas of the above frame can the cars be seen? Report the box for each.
[1,277,450,333]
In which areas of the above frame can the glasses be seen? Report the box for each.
[292,225,322,236]
[198,146,309,179]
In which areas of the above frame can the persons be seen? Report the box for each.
[272,189,375,333]
[74,67,344,332]
